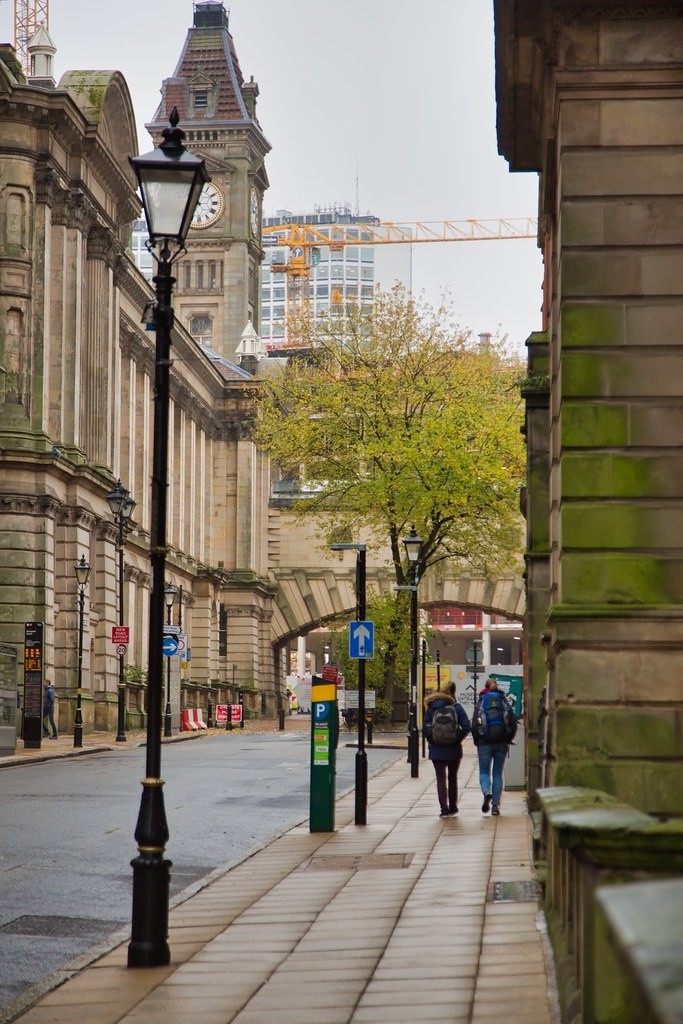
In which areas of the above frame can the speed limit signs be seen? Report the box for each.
[116,644,127,656]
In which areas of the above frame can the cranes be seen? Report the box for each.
[271,212,543,350]
[13,0,51,75]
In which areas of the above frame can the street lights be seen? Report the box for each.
[404,525,423,777]
[104,479,137,740]
[74,554,92,748]
[329,540,372,828]
[161,581,178,737]
[473,639,485,708]
[122,107,207,963]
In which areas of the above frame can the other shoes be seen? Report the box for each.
[42,731,50,737]
[49,735,57,739]
[440,806,459,816]
[492,807,500,814]
[482,793,492,812]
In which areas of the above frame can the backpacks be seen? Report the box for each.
[426,702,461,745]
[476,692,511,738]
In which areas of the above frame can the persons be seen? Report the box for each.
[17,682,21,738]
[471,678,518,816]
[423,681,471,819]
[42,679,57,740]
